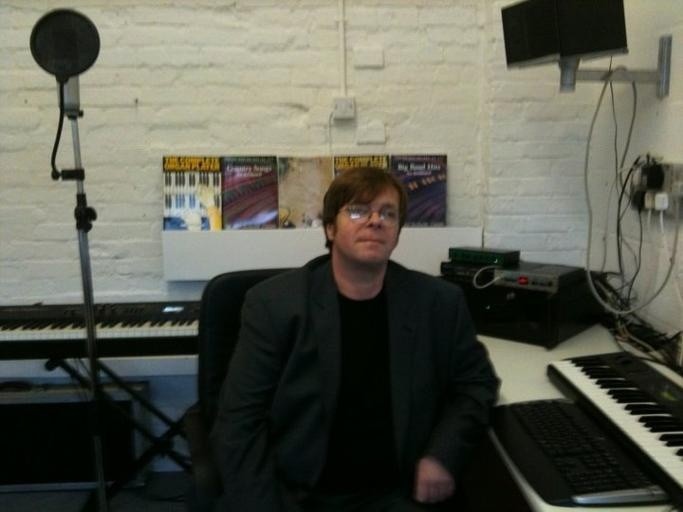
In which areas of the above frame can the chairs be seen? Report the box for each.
[186,264,293,509]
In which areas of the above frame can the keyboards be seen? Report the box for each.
[489,400,672,507]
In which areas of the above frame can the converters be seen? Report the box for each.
[647,163,664,191]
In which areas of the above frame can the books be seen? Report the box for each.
[219,154,279,231]
[160,155,222,231]
[389,153,447,227]
[333,155,389,178]
[276,155,333,230]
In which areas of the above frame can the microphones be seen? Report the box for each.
[55,6,82,115]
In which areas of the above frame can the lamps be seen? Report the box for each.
[497,2,674,101]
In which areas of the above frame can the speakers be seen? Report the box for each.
[500,0,629,72]
[0,381,151,494]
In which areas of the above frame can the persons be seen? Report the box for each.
[207,167,502,510]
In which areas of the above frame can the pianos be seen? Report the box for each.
[0,303,201,360]
[547,351,683,509]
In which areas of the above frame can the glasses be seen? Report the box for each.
[337,202,404,228]
